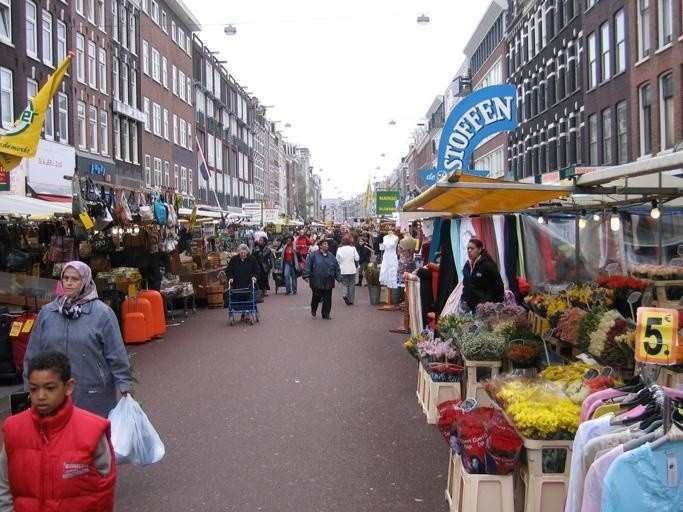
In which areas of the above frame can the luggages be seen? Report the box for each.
[0,315,19,384]
[119,287,152,345]
[11,313,46,375]
[122,296,146,344]
[134,278,168,339]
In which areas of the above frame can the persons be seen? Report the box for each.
[295,229,313,260]
[378,230,399,289]
[320,226,333,239]
[251,236,275,296]
[293,231,300,246]
[553,243,573,284]
[1,349,117,512]
[336,237,360,305]
[279,235,301,295]
[274,237,286,251]
[306,234,320,259]
[333,228,344,244]
[302,239,341,319]
[344,227,361,240]
[396,232,417,287]
[22,260,134,419]
[356,238,369,286]
[461,238,504,315]
[225,243,260,321]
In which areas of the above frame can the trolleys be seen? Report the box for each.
[228,280,260,326]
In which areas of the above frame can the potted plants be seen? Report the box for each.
[439,312,502,397]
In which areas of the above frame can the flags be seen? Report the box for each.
[364,178,373,208]
[0,56,72,172]
[196,140,211,180]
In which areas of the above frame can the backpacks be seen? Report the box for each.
[163,201,177,228]
[116,189,133,224]
[155,201,167,225]
[127,190,141,222]
[140,206,154,224]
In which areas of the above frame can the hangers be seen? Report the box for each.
[620,367,683,442]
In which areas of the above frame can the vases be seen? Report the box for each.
[527,312,550,335]
[413,364,462,423]
[370,286,380,305]
[444,448,515,512]
[503,411,574,512]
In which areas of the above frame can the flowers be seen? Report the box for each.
[631,265,683,298]
[562,277,610,309]
[403,332,463,382]
[526,294,569,320]
[365,262,382,285]
[492,380,582,470]
[579,306,635,366]
[594,275,647,308]
[437,399,521,471]
[555,307,583,342]
[477,291,526,331]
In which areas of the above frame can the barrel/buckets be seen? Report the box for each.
[207,286,224,308]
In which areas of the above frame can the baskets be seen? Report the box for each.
[207,252,220,267]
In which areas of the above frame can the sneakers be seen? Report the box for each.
[349,302,353,304]
[343,296,349,303]
[322,314,331,320]
[311,311,316,316]
[293,291,296,295]
[285,290,291,295]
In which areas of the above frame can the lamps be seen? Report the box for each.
[609,208,620,231]
[578,210,587,229]
[651,198,661,218]
[536,214,544,223]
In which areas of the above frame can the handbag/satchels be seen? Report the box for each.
[48,222,74,262]
[31,263,42,278]
[52,224,79,277]
[79,179,177,229]
[6,223,32,272]
